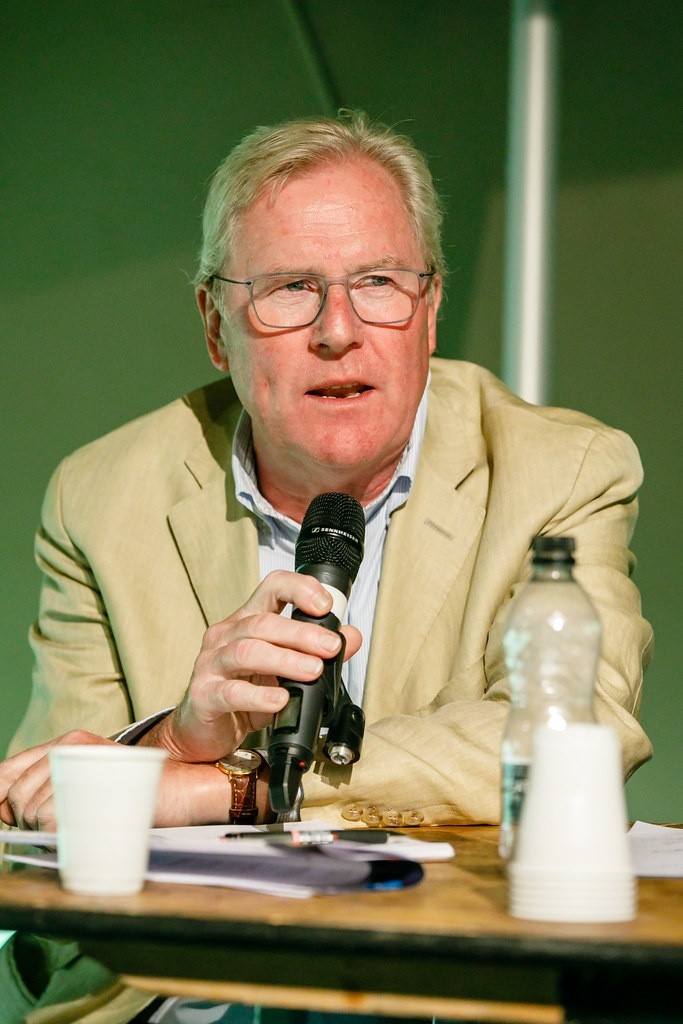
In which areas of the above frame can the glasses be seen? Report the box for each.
[211,265,436,328]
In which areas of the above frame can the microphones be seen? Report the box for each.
[266,492,367,813]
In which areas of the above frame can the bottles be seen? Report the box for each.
[500,535,604,858]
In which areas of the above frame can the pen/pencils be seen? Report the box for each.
[219,829,390,845]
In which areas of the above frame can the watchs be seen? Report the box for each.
[218,750,266,828]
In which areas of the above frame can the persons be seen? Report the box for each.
[0,110,654,1024]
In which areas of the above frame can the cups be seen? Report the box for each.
[45,744,165,897]
[511,724,635,920]
